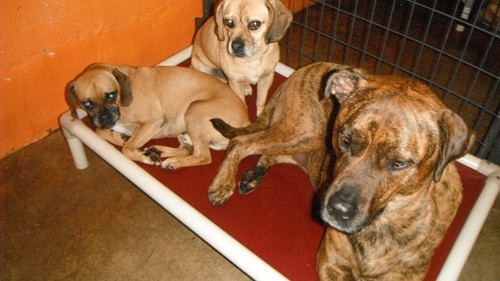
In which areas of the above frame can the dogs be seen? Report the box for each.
[207,62,477,281]
[187,0,294,119]
[65,62,250,171]
